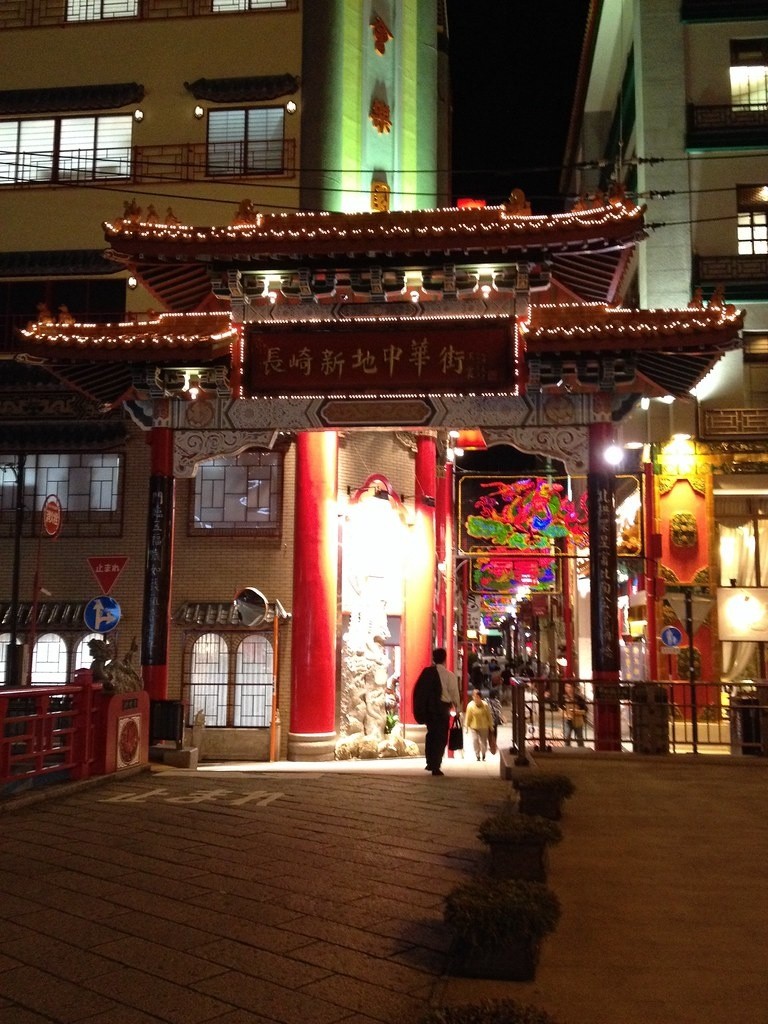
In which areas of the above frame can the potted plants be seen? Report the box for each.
[510,767,578,820]
[477,814,565,882]
[442,875,564,984]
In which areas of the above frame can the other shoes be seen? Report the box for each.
[425,764,432,771]
[476,757,480,761]
[432,769,444,775]
[483,756,485,761]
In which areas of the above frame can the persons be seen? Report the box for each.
[464,645,588,763]
[413,648,462,776]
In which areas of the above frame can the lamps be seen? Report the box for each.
[134,109,143,122]
[194,105,204,119]
[128,276,138,290]
[286,99,297,115]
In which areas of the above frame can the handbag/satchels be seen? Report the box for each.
[448,715,463,750]
[495,716,503,725]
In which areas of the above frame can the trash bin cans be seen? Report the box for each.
[631,684,671,754]
